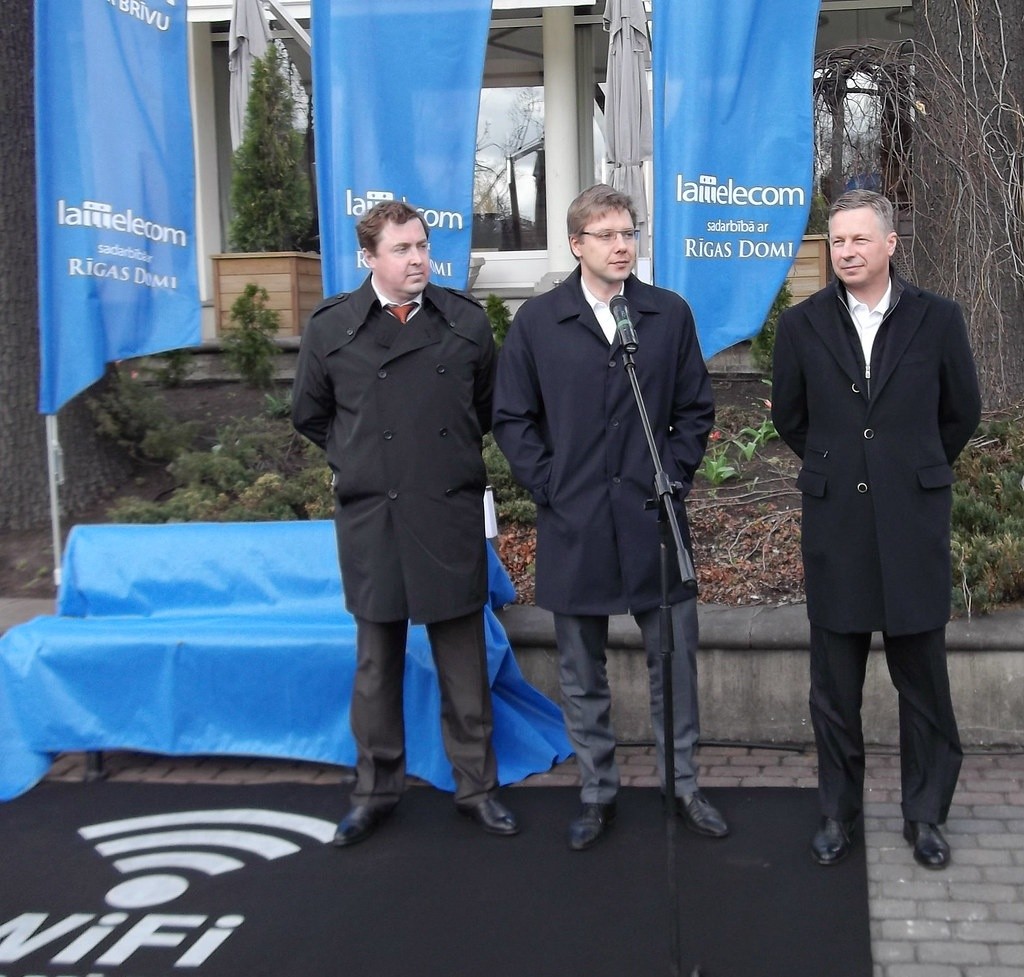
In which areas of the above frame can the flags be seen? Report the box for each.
[30,0,206,416]
[651,1,822,363]
[311,0,495,304]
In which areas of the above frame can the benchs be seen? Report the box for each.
[1,517,582,804]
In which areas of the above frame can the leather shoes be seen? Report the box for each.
[902,820,950,870]
[456,799,521,835]
[809,814,858,866]
[332,803,397,847]
[660,789,730,838]
[567,803,618,850]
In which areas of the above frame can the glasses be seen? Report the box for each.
[580,228,640,244]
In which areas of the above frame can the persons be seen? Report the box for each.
[290,202,517,845]
[773,187,983,869]
[492,185,727,852]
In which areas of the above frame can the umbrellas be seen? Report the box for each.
[533,149,547,248]
[228,1,273,157]
[604,1,652,281]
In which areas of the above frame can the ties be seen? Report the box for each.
[383,302,418,326]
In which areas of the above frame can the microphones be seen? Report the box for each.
[610,294,639,355]
